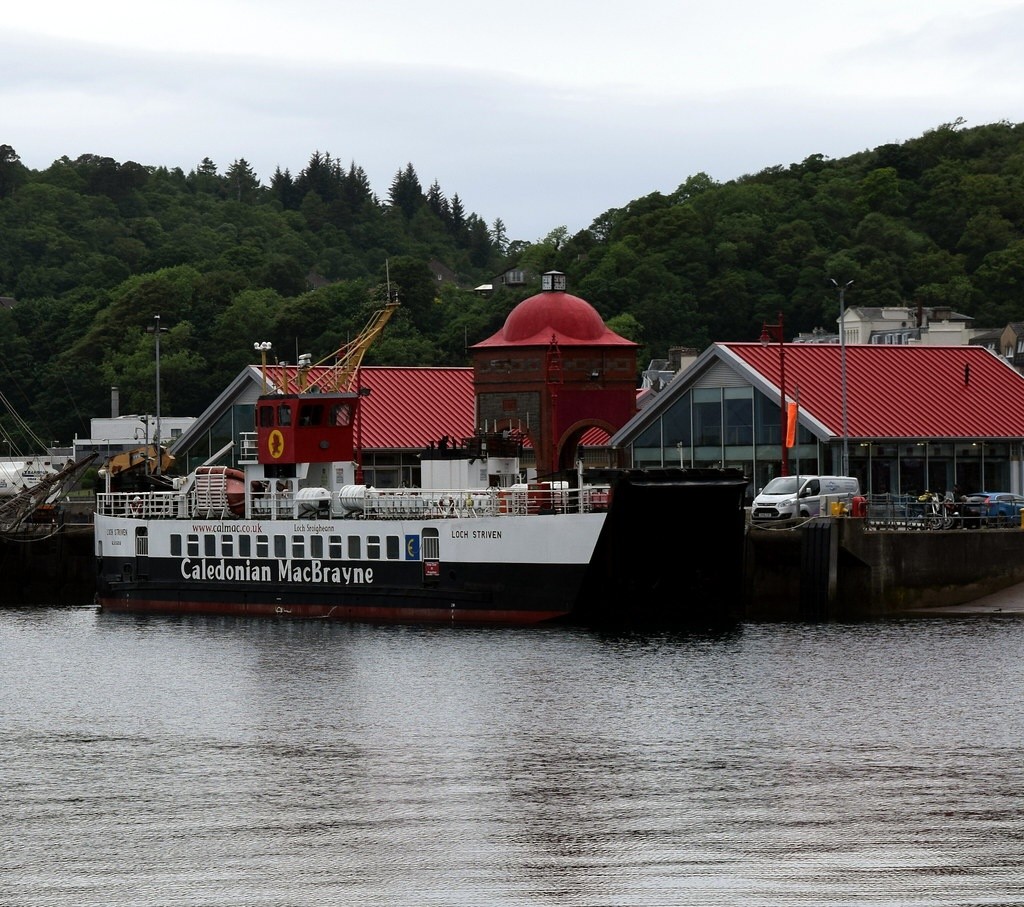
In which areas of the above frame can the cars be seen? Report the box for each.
[962,492,1024,527]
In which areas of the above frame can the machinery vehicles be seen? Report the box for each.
[0,461,61,523]
[94,388,747,626]
[91,444,179,515]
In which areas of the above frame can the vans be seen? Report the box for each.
[751,476,862,524]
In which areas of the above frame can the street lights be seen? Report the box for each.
[831,278,855,476]
[50,441,59,463]
[3,440,11,461]
[918,441,928,491]
[972,441,984,492]
[147,314,167,475]
[103,439,109,455]
[860,442,872,507]
[295,327,307,379]
[254,340,271,393]
[760,324,788,477]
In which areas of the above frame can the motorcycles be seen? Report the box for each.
[901,484,959,530]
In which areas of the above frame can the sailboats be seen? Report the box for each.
[0,391,93,605]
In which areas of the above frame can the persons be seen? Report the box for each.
[128,496,142,519]
[951,485,974,529]
